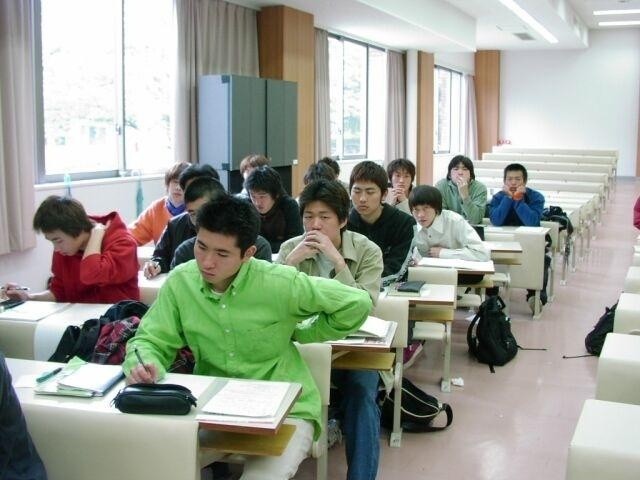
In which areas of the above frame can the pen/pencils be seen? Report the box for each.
[35,366,62,382]
[148,262,160,280]
[134,348,152,376]
[1,287,29,291]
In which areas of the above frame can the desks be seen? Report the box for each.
[480,241,525,254]
[0,299,400,352]
[5,356,303,436]
[139,270,456,306]
[411,258,496,276]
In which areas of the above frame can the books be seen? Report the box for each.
[32,356,124,398]
[347,316,392,338]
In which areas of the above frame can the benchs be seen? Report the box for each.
[613,293,640,336]
[455,241,493,316]
[19,403,202,479]
[485,234,523,316]
[563,398,640,480]
[136,246,459,394]
[138,288,410,448]
[624,265,639,294]
[0,320,332,480]
[460,144,620,259]
[632,245,640,267]
[596,333,639,406]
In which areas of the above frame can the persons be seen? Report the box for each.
[295,157,350,205]
[403,184,492,285]
[121,193,373,480]
[126,161,192,248]
[0,194,142,304]
[142,162,221,281]
[274,178,385,480]
[234,153,304,255]
[346,160,424,370]
[168,176,274,272]
[433,155,487,241]
[382,157,416,216]
[0,351,49,480]
[485,163,551,315]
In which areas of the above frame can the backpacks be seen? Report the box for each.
[466,294,548,374]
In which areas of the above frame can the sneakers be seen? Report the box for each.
[402,340,423,369]
[527,296,542,314]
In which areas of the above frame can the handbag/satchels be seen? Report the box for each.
[380,376,453,433]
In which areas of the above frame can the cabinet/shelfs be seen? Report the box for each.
[195,75,266,193]
[266,77,298,200]
